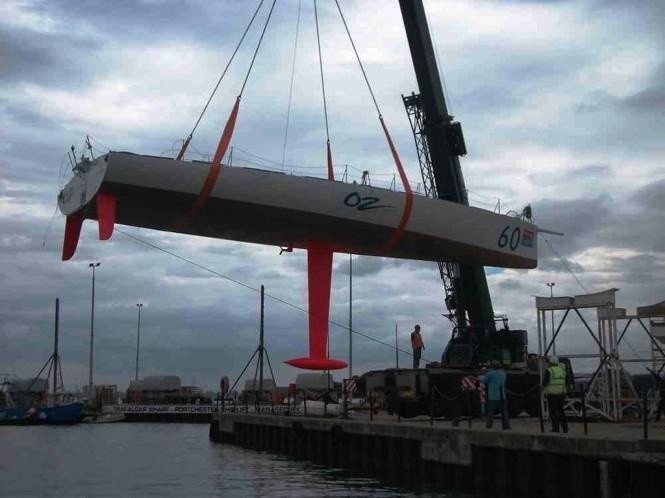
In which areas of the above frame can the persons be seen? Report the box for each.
[410,325,426,369]
[543,354,570,434]
[468,359,513,430]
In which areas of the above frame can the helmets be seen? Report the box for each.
[548,356,559,365]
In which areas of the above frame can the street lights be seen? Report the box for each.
[86,261,100,400]
[547,282,556,356]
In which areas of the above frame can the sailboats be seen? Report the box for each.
[0,283,339,427]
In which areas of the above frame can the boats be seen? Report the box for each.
[53,134,567,372]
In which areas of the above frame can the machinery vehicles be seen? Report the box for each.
[388,0,577,420]
